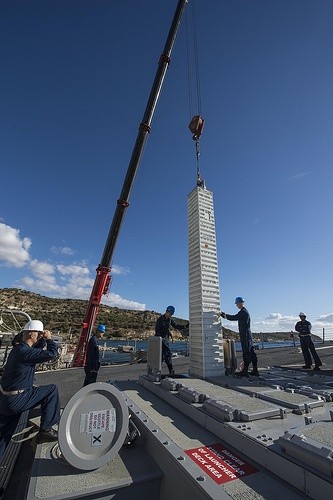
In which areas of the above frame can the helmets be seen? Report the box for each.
[299,312,307,317]
[166,306,175,314]
[22,320,43,331]
[97,325,106,333]
[234,297,245,304]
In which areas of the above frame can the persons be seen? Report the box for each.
[295,312,323,370]
[84,324,105,386]
[155,306,189,376]
[220,297,259,376]
[0,320,60,460]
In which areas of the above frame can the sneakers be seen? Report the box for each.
[37,428,57,444]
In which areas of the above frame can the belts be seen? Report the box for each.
[0,384,24,395]
[300,334,311,337]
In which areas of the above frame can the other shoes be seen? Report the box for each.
[314,366,320,370]
[249,370,259,376]
[302,366,311,369]
[234,370,248,377]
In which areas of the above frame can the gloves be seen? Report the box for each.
[221,311,225,318]
[185,323,190,328]
